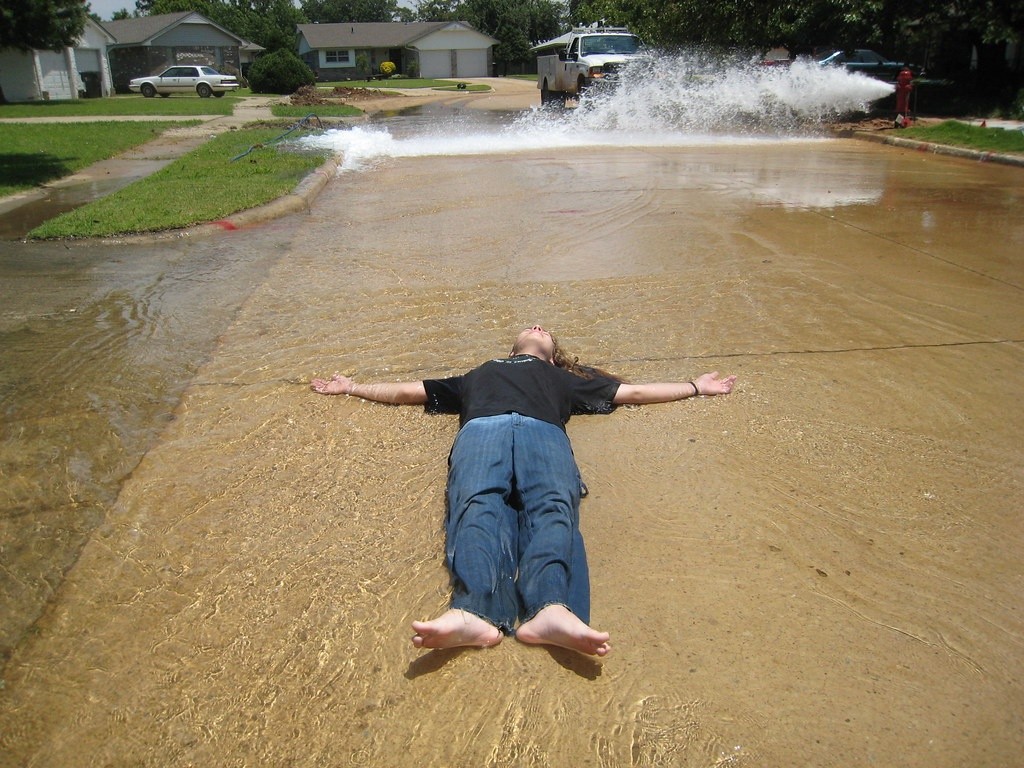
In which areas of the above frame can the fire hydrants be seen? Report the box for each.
[893,70,913,128]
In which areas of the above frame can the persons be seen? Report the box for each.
[310,324,736,656]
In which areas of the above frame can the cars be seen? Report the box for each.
[757,47,793,71]
[801,45,928,85]
[129,65,240,99]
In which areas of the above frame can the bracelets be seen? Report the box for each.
[689,382,698,396]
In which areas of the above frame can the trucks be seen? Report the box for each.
[537,24,657,113]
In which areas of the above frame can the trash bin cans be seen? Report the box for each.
[79,71,102,98]
